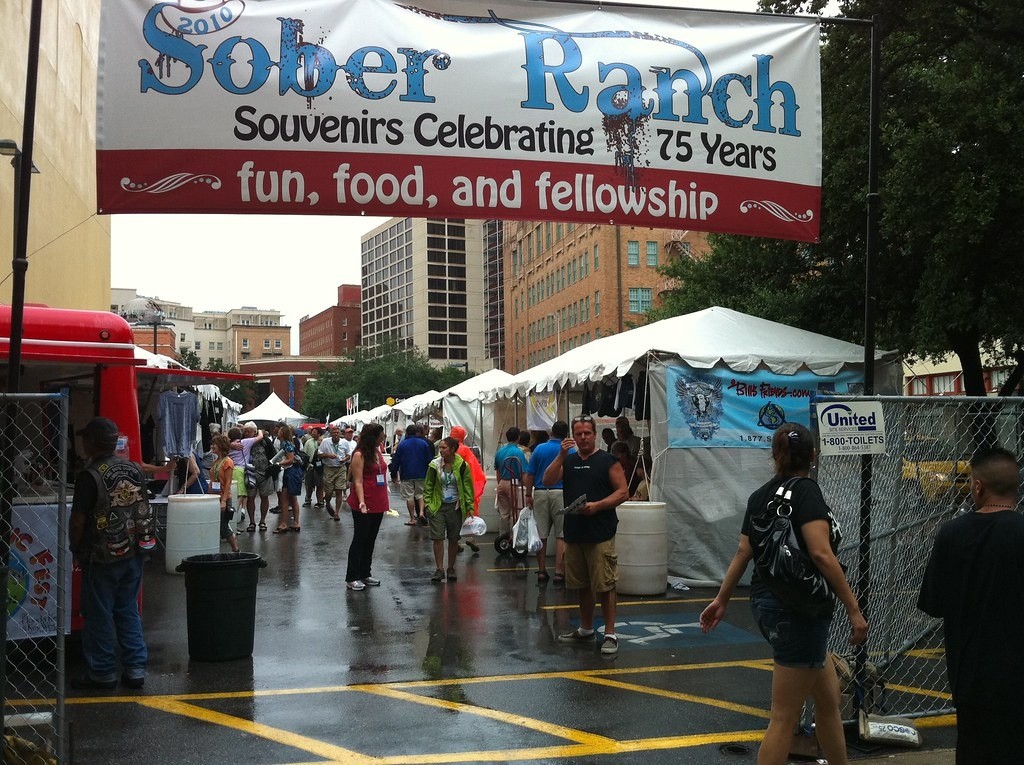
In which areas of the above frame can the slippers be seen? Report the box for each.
[404,522,416,525]
[288,527,300,531]
[273,528,287,532]
[420,517,428,524]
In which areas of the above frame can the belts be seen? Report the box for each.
[534,487,564,490]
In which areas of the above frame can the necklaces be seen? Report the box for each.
[982,503,1013,508]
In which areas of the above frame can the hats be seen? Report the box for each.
[76,416,119,442]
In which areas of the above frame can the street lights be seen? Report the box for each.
[135,321,174,355]
[452,363,468,380]
[547,313,560,356]
[2,139,40,258]
[469,356,479,376]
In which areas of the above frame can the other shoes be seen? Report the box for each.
[268,499,342,520]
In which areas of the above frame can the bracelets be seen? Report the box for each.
[69,546,79,553]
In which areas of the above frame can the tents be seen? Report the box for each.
[330,369,515,475]
[477,306,904,590]
[237,391,308,429]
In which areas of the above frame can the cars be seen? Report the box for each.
[900,434,974,509]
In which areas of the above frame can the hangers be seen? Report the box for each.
[169,382,184,394]
[604,370,619,383]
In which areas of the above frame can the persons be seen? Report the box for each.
[68,417,157,690]
[698,422,868,765]
[917,448,1024,765]
[345,423,390,591]
[379,418,652,582]
[542,414,629,655]
[142,421,360,554]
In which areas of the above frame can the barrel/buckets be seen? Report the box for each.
[615,501,668,595]
[165,494,221,574]
[208,480,238,542]
[478,475,500,533]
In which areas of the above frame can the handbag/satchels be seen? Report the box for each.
[512,506,543,551]
[245,465,256,488]
[459,515,486,536]
[294,455,304,466]
[751,476,848,624]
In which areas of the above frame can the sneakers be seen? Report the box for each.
[466,537,480,552]
[447,568,456,578]
[347,579,366,590]
[457,544,464,551]
[362,576,380,585]
[431,569,445,579]
[558,629,597,643]
[601,636,618,653]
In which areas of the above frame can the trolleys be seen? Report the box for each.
[494,455,528,557]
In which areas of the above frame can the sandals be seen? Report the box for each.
[259,523,267,531]
[538,571,550,580]
[553,573,565,582]
[247,524,256,531]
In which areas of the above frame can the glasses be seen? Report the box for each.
[573,416,593,421]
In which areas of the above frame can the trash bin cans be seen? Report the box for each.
[182,552,262,663]
